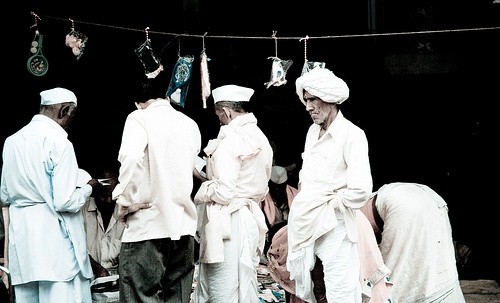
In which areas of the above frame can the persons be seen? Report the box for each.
[288,68,373,303]
[360,183,467,303]
[112,71,201,303]
[0,87,98,303]
[263,167,298,264]
[194,85,274,303]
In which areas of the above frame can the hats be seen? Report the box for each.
[40,88,77,107]
[271,166,288,184]
[212,85,255,104]
[296,69,350,106]
